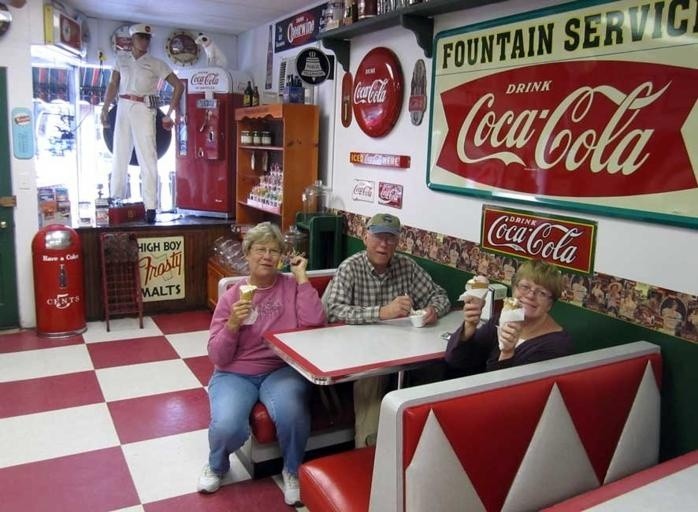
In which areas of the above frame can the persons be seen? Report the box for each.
[445,259,573,379]
[321,214,450,449]
[102,23,185,224]
[199,220,328,506]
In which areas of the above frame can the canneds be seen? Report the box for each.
[241,130,272,146]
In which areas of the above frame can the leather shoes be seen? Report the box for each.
[146,211,156,224]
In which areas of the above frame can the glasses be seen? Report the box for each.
[514,281,553,302]
[367,231,398,246]
[248,246,281,256]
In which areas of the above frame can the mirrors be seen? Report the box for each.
[28,59,77,224]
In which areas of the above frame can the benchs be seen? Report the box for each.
[218,268,355,480]
[299,341,663,511]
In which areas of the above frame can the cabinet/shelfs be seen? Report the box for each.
[293,209,346,270]
[70,208,236,320]
[205,104,320,306]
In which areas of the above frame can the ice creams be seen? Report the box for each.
[502,296,520,311]
[239,285,257,301]
[466,275,489,289]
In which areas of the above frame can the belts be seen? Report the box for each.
[118,94,146,104]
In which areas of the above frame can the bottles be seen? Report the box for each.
[239,130,273,146]
[319,0,431,33]
[302,179,337,225]
[249,162,283,208]
[280,224,311,267]
[241,73,304,108]
[263,23,275,90]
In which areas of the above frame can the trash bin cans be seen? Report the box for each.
[31,224,87,339]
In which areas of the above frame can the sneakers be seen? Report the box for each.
[281,468,305,509]
[197,459,222,494]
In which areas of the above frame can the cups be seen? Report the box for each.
[146,209,156,221]
[409,311,428,328]
[77,201,91,223]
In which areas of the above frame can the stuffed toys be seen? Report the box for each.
[194,32,229,67]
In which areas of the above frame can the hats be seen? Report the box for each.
[129,24,152,39]
[365,212,401,237]
